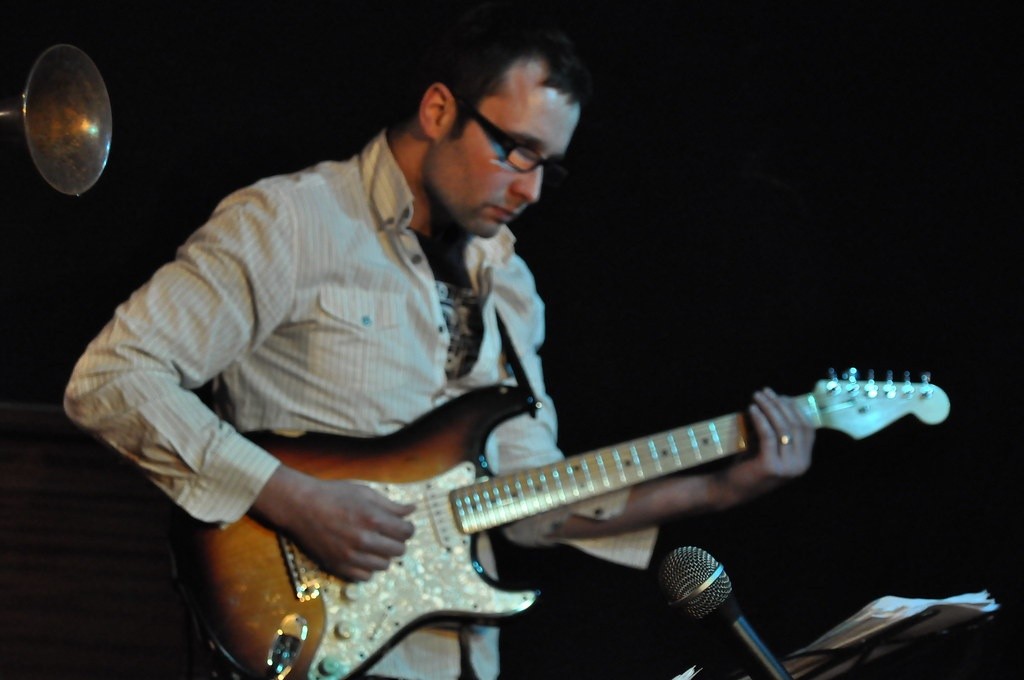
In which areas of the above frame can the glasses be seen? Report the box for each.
[470,110,567,181]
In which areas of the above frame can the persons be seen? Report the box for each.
[63,0,815,680]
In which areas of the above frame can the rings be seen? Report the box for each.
[779,435,793,446]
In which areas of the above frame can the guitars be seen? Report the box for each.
[165,367,952,680]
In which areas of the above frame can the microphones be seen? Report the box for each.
[658,546,794,680]
